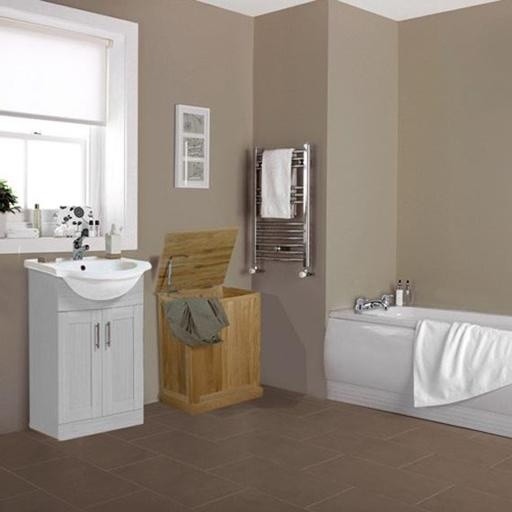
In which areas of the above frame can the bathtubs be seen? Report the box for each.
[323,305,512,438]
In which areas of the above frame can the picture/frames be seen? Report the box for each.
[174,103,210,190]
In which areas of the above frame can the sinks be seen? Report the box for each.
[59,258,142,301]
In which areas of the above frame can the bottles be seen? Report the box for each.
[89,220,100,237]
[394,280,403,306]
[403,280,411,306]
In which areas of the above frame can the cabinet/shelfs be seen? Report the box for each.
[26,303,144,442]
[246,142,314,279]
[154,228,264,416]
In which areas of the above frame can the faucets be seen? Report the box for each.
[365,298,388,311]
[72,234,89,260]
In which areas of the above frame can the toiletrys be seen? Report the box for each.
[393,280,405,306]
[401,279,413,306]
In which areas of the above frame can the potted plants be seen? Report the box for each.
[0,179,22,239]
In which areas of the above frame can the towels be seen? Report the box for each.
[258,147,297,219]
[412,320,512,409]
